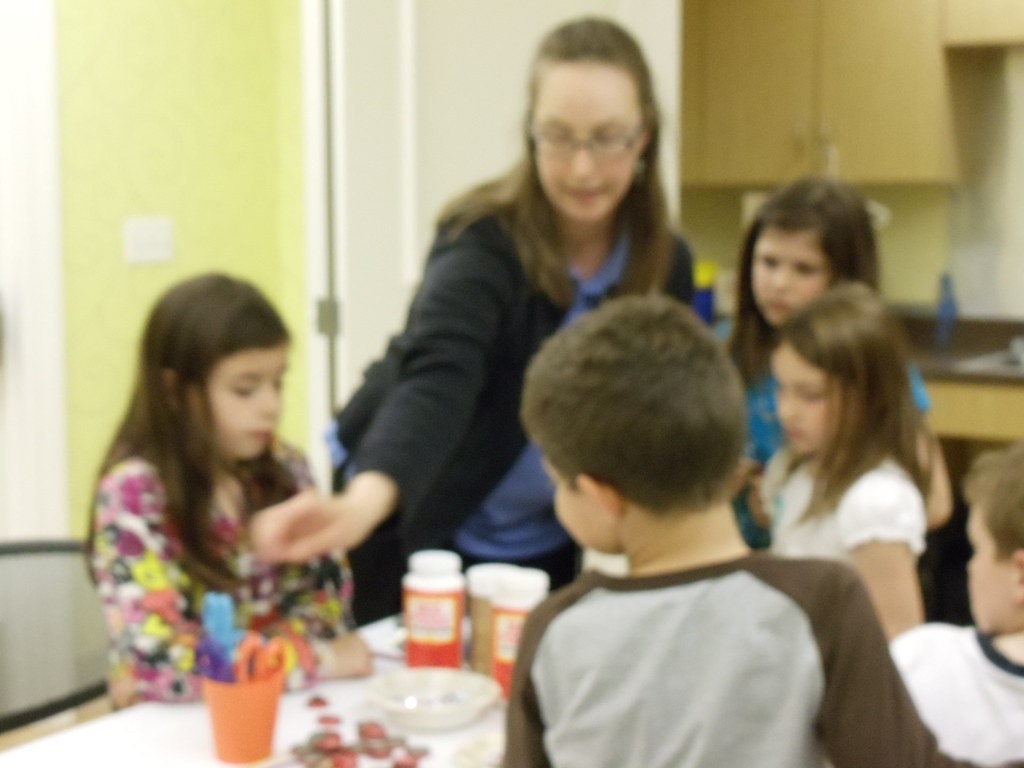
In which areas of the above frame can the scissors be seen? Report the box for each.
[200,590,296,685]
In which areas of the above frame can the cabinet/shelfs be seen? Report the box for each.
[681,0,1005,191]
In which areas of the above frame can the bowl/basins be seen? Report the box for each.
[367,668,501,730]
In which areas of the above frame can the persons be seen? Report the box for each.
[713,177,955,554]
[248,18,696,628]
[760,300,942,643]
[83,275,374,715]
[500,295,967,768]
[886,442,1023,768]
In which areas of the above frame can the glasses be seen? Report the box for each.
[533,124,642,159]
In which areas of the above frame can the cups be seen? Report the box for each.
[195,667,282,763]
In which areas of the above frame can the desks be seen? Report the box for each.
[0,614,506,768]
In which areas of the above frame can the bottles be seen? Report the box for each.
[489,567,550,698]
[401,549,466,667]
[468,562,513,674]
[927,274,960,353]
